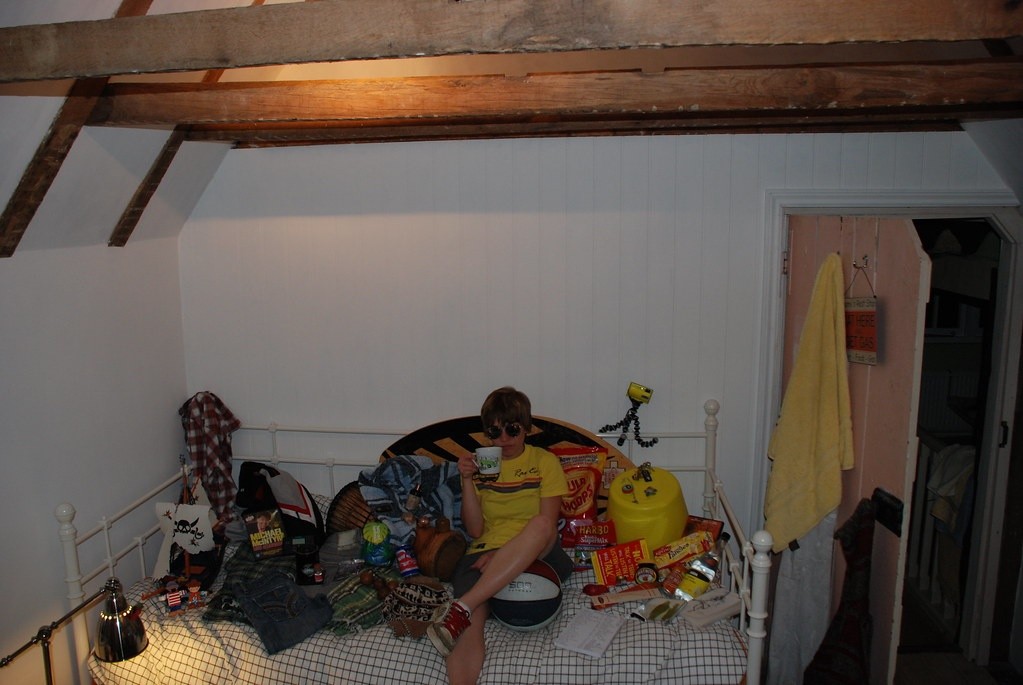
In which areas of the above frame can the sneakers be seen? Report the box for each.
[426,599,472,657]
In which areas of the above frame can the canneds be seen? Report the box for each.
[662,564,689,595]
[394,543,420,578]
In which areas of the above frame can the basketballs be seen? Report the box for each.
[489,557,563,632]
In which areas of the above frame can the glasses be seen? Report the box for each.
[484,423,522,440]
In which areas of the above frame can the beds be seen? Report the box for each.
[56,390,772,685]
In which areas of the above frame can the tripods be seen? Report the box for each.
[599,396,659,448]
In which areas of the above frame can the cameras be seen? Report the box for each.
[626,382,653,405]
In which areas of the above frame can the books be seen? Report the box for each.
[678,589,745,628]
[555,606,626,658]
[246,508,289,560]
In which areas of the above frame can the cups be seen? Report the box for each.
[471,447,503,474]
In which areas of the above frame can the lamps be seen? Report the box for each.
[0,577,149,685]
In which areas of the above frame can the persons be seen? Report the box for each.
[427,387,568,685]
[159,579,207,616]
[257,512,271,533]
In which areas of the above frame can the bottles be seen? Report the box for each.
[404,481,421,511]
[674,532,731,602]
